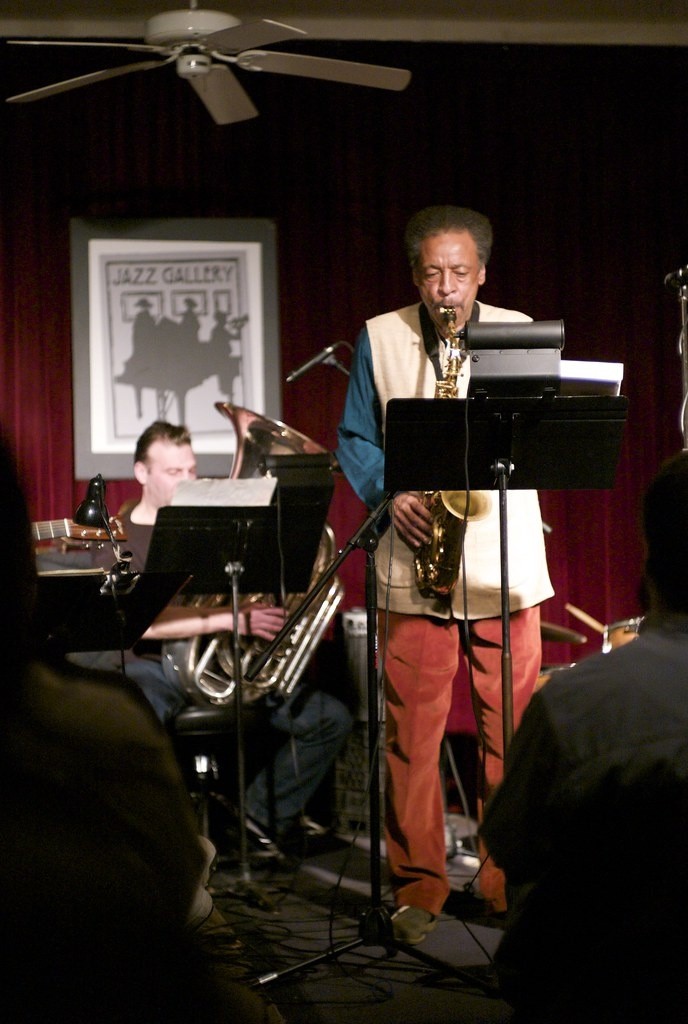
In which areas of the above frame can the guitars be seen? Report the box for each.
[33,512,128,546]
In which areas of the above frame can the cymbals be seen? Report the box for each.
[539,621,588,644]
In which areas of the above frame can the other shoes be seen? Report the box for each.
[227,801,287,866]
[486,909,508,927]
[390,905,438,944]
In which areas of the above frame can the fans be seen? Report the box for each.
[5,1,411,126]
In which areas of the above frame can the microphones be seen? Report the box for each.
[282,342,341,383]
[663,263,688,295]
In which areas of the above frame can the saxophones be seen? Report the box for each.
[414,306,493,601]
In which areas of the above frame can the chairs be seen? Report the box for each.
[172,701,293,874]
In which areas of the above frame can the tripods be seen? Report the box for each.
[143,492,501,998]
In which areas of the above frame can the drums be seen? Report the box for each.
[533,664,580,694]
[601,617,643,654]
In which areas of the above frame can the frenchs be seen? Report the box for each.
[162,401,347,710]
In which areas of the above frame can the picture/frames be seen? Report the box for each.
[70,217,282,481]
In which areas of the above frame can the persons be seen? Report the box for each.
[85,421,355,866]
[335,207,555,945]
[476,453,688,1024]
[0,430,267,1024]
